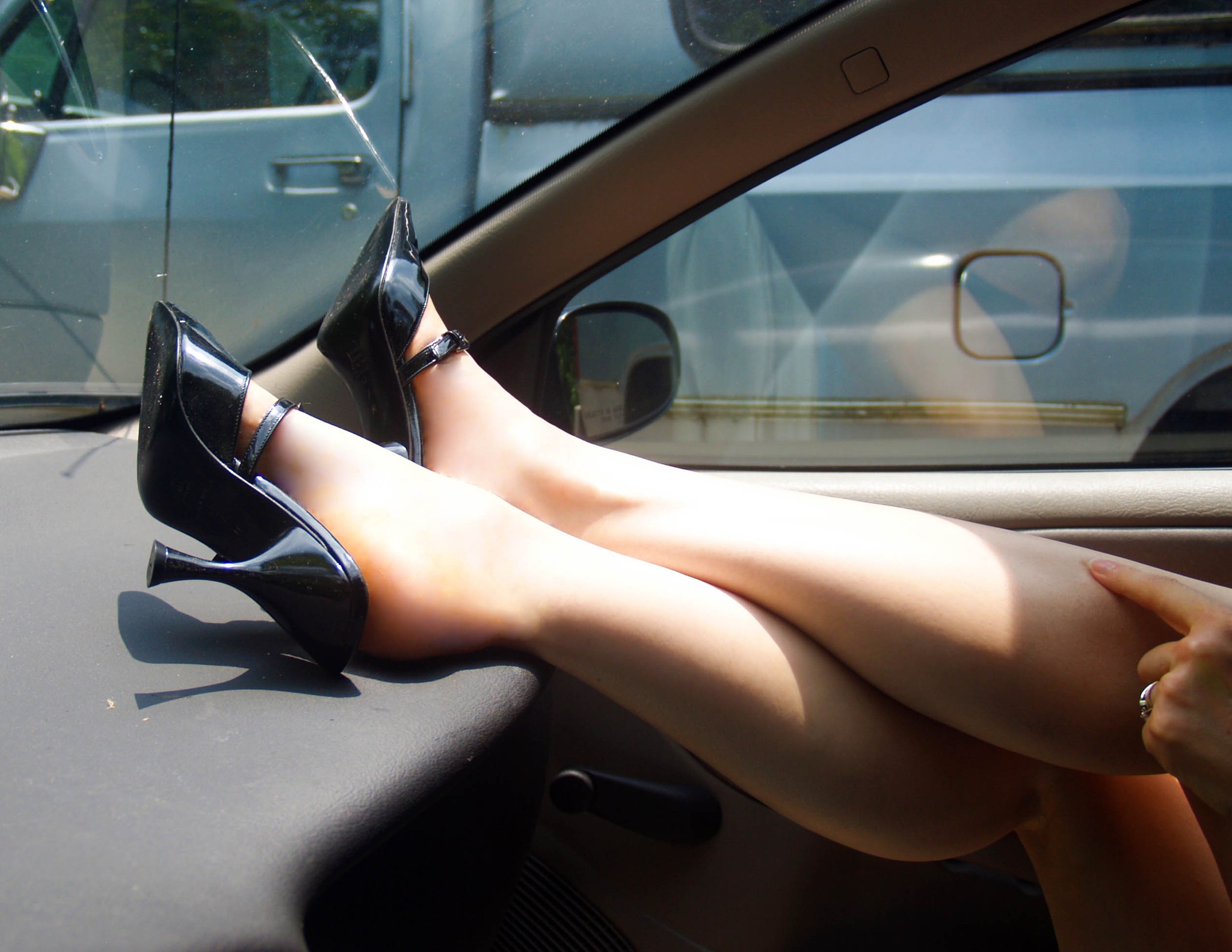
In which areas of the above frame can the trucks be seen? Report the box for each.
[0,0,1232,472]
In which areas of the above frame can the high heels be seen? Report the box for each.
[139,300,370,675]
[315,199,470,465]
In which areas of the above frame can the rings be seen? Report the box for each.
[1138,682,1158,719]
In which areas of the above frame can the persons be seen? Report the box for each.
[139,196,1231,949]
